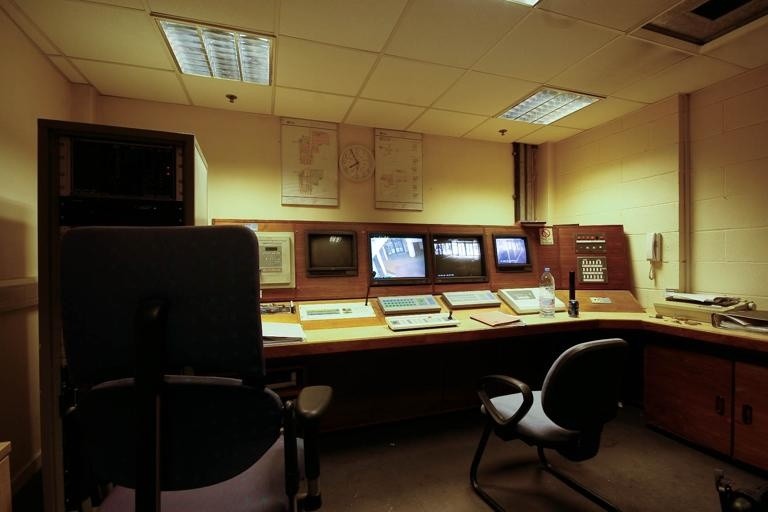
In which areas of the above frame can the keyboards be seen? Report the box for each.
[378,295,441,315]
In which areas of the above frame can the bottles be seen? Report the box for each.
[537,267,556,319]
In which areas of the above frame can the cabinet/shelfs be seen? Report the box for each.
[644,313,766,474]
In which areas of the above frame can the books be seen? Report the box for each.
[711,308,768,333]
[519,221,546,227]
[470,310,521,327]
[263,321,304,344]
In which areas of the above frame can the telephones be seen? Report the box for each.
[646,232,663,280]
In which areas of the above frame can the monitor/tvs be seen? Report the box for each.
[432,233,488,284]
[368,232,432,285]
[494,233,532,273]
[303,230,358,277]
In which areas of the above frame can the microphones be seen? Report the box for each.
[366,271,376,305]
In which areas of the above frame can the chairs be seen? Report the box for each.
[469,338,633,491]
[56,225,331,510]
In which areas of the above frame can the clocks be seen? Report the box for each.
[340,143,375,182]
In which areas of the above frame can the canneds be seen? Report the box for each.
[568,301,579,316]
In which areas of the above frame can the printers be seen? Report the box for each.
[653,293,756,323]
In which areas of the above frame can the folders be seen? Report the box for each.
[261,322,303,344]
[711,309,767,330]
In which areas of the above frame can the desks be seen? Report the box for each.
[261,308,644,448]
